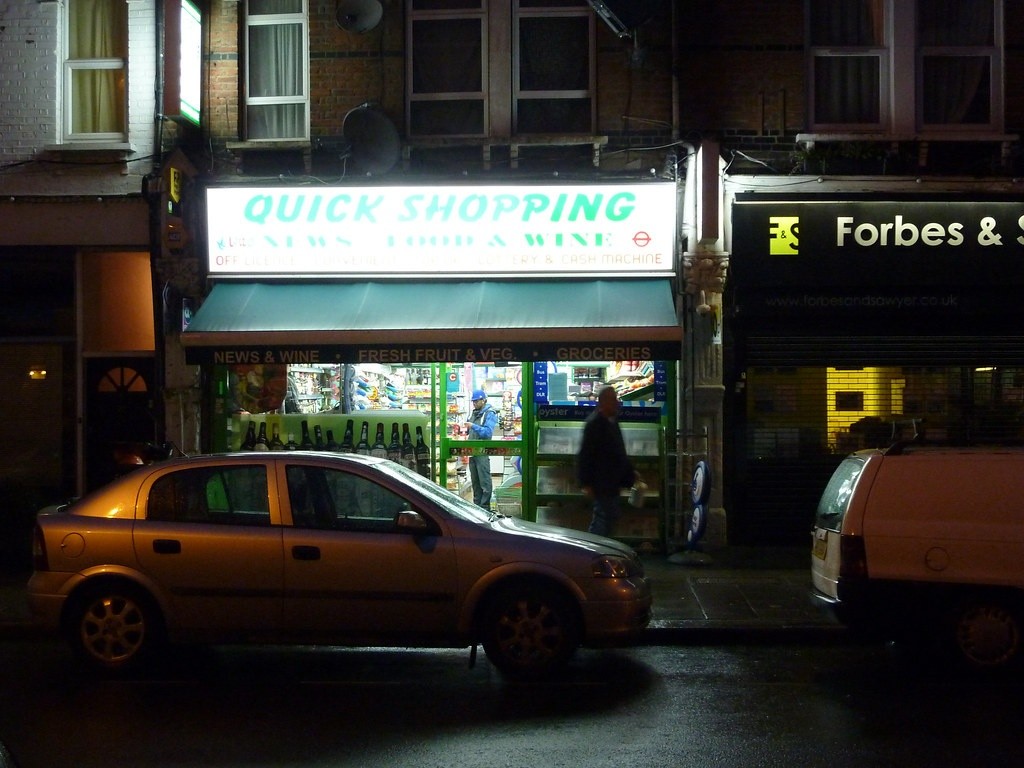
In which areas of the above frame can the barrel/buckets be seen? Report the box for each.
[628,478,648,508]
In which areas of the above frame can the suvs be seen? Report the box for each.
[807,434,1024,682]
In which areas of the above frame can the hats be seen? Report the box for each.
[469,390,485,400]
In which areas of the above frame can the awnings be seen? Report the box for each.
[179,282,683,363]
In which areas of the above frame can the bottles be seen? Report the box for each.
[402,423,415,471]
[388,423,402,465]
[211,364,234,453]
[240,421,270,451]
[325,430,341,451]
[371,423,388,459]
[270,423,283,450]
[356,421,371,455]
[300,420,315,451]
[285,434,300,450]
[314,425,328,451]
[414,426,430,479]
[339,419,356,454]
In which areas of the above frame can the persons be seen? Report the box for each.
[464,390,498,512]
[577,387,641,536]
[111,429,148,484]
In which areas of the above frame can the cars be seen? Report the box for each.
[23,451,654,680]
